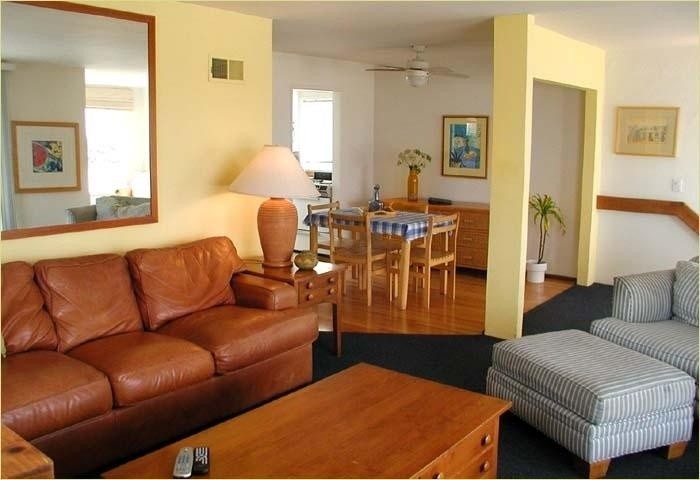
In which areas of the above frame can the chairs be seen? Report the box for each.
[304,198,463,312]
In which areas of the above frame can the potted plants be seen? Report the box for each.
[521,192,569,286]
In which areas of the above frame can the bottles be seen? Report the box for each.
[368,191,384,212]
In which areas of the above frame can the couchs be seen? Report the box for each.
[62,193,152,225]
[589,255,699,423]
[0,234,322,479]
[484,322,699,479]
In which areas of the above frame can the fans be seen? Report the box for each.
[361,42,475,80]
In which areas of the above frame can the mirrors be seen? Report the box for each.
[1,0,161,241]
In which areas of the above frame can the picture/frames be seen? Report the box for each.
[9,118,84,194]
[612,103,680,160]
[439,113,489,180]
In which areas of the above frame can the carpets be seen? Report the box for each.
[52,283,699,478]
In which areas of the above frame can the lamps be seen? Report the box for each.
[401,71,430,87]
[227,144,321,270]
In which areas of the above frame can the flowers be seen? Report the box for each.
[394,147,433,175]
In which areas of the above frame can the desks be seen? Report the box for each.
[237,249,350,360]
[1,417,55,479]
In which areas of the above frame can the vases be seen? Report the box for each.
[405,167,420,203]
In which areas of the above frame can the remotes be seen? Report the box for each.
[194,447,209,475]
[173,446,194,478]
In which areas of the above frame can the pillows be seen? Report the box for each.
[91,194,151,222]
[669,258,700,330]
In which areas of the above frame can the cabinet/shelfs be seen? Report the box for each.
[364,194,489,275]
